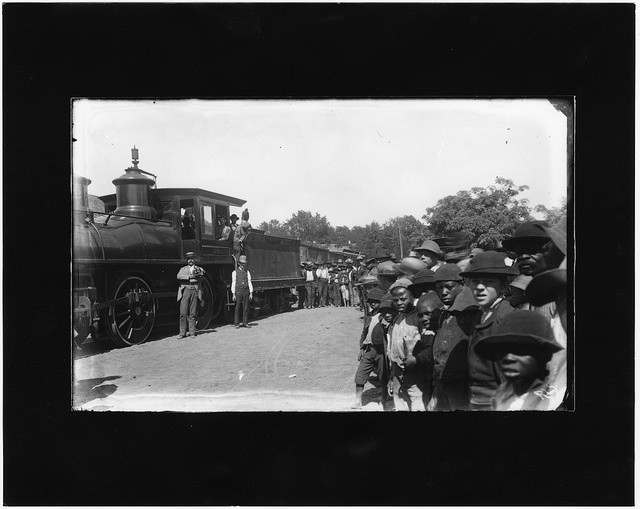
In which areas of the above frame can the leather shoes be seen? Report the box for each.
[177,336,184,339]
[235,324,239,328]
[190,335,195,338]
[245,324,250,328]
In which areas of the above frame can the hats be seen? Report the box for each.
[230,214,239,219]
[367,287,383,301]
[501,220,551,250]
[414,240,444,257]
[370,261,401,276]
[458,251,521,277]
[237,254,249,264]
[407,270,437,290]
[525,270,569,307]
[433,263,462,284]
[389,277,413,294]
[372,248,392,258]
[216,213,228,221]
[392,257,429,277]
[184,251,197,259]
[377,295,394,312]
[471,311,563,362]
[354,276,379,286]
[355,254,366,261]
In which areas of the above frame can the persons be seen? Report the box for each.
[381,300,397,364]
[299,259,397,309]
[416,240,442,271]
[227,214,245,262]
[182,206,196,239]
[471,308,565,412]
[433,264,476,411]
[459,250,520,410]
[214,216,231,244]
[445,252,462,263]
[409,269,434,295]
[501,220,566,316]
[352,289,387,408]
[177,251,205,338]
[526,256,568,341]
[387,280,428,411]
[231,255,254,328]
[401,293,447,379]
[399,257,428,277]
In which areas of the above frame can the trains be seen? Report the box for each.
[72,144,360,348]
